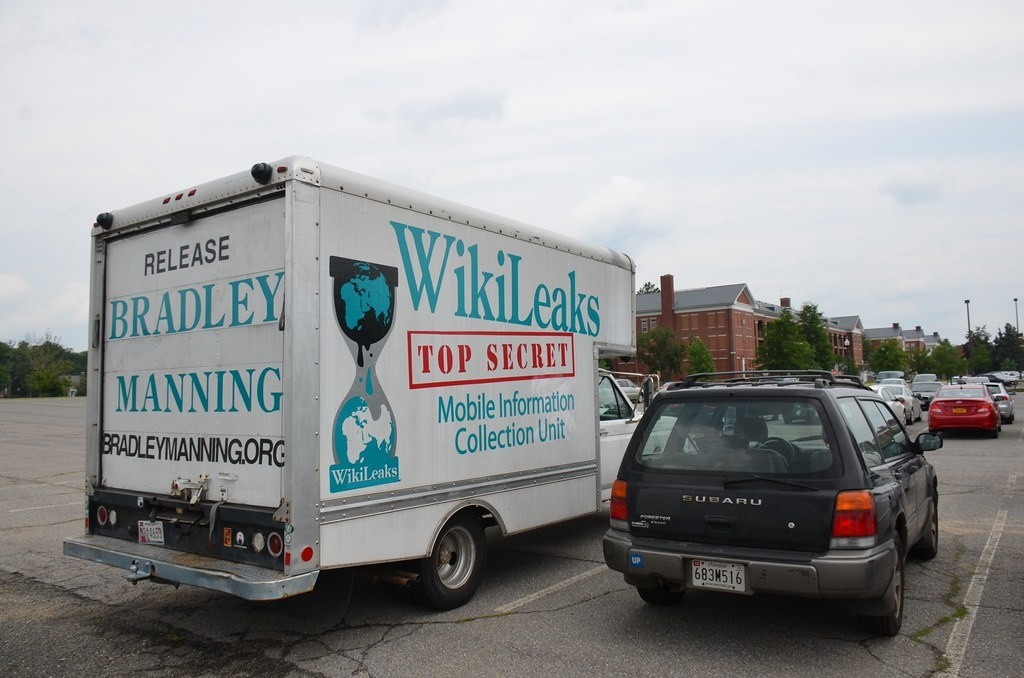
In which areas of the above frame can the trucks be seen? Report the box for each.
[59,153,651,614]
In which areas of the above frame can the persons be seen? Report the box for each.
[957,375,966,384]
[731,417,789,472]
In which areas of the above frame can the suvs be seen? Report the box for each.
[603,369,948,636]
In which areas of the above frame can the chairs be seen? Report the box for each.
[810,420,882,472]
[698,449,789,472]
[734,417,768,442]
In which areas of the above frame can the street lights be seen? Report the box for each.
[964,298,974,372]
[1013,297,1020,336]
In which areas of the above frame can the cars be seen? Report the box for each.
[927,384,1002,438]
[873,367,1024,427]
[984,382,1017,425]
[656,381,684,411]
[615,378,643,405]
